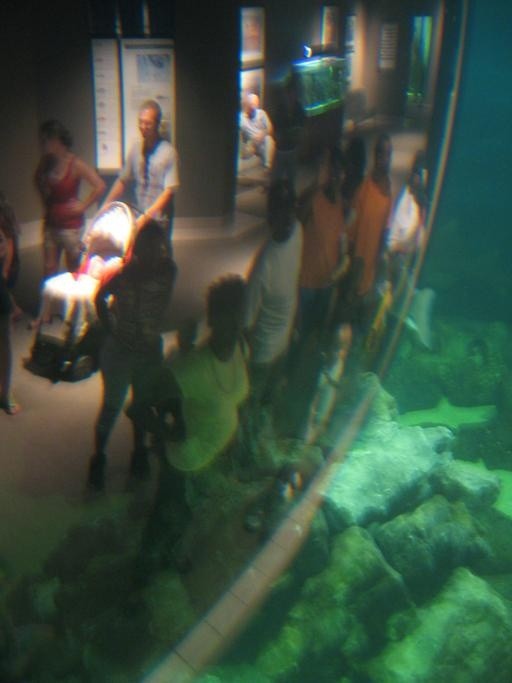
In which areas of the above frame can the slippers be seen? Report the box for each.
[0,393,21,415]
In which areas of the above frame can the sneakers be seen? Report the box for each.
[243,500,272,532]
[88,452,106,492]
[131,446,150,480]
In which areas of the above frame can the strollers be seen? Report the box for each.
[26,196,166,382]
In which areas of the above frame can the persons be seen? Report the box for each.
[0,191,24,415]
[25,115,109,329]
[80,95,182,266]
[31,251,108,335]
[236,89,274,171]
[84,128,438,586]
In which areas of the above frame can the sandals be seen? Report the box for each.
[27,312,53,329]
[166,538,193,575]
[9,306,25,322]
